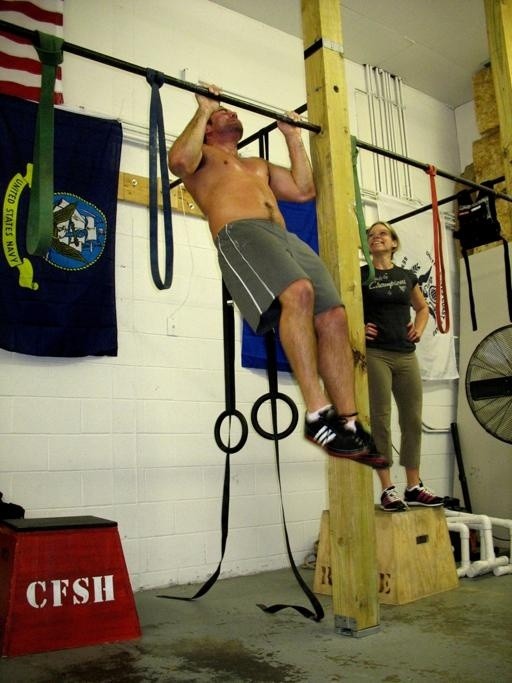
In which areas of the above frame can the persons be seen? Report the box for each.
[165,81,392,472]
[359,221,446,513]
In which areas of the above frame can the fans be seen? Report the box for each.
[465,324,512,445]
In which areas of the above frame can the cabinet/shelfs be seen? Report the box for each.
[454,241,512,555]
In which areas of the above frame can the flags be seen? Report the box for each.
[241,196,319,373]
[372,189,460,383]
[0,0,66,104]
[0,91,124,358]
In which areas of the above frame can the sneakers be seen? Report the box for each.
[379,483,408,512]
[302,405,371,459]
[402,483,450,507]
[349,419,391,470]
